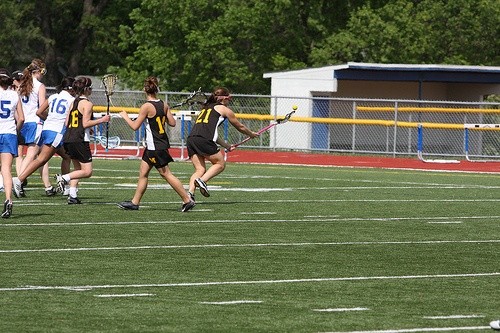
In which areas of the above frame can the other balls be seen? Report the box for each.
[292,104,298,110]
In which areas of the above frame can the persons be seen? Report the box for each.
[187,87,260,207]
[116,76,196,212]
[0,59,110,219]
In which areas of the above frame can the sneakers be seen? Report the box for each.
[181,198,195,212]
[45,185,56,196]
[0,186,6,193]
[194,177,210,197]
[12,176,25,199]
[182,192,195,209]
[62,186,79,196]
[67,195,81,204]
[117,200,140,210]
[54,174,68,194]
[0,199,13,219]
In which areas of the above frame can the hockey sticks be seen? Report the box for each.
[100,73,118,153]
[223,110,296,153]
[130,87,210,121]
[89,136,120,149]
